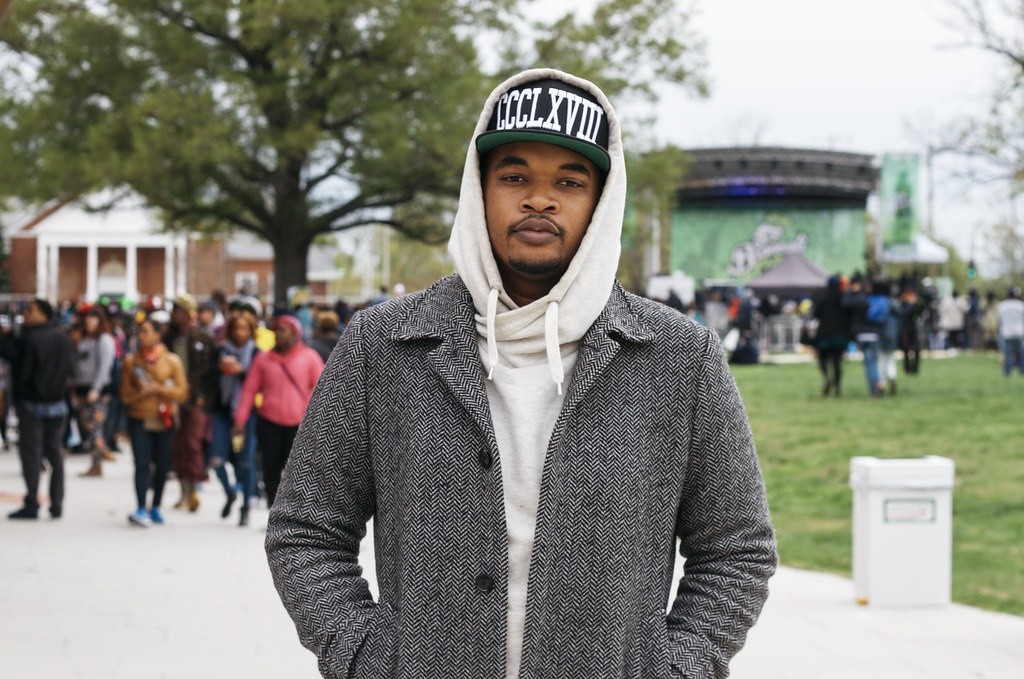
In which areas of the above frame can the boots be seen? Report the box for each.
[175,486,200,512]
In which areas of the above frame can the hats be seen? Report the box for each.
[474,78,612,174]
[170,291,196,317]
[275,315,303,341]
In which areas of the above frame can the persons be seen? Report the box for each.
[120,321,189,525]
[216,312,262,527]
[76,311,118,475]
[161,295,216,511]
[264,69,777,679]
[669,265,1024,396]
[231,316,324,509]
[0,286,389,454]
[8,299,79,518]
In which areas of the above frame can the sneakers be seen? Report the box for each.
[128,506,164,527]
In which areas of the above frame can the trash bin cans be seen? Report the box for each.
[847,452,958,611]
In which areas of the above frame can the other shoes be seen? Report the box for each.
[878,384,885,396]
[222,492,237,517]
[239,506,249,526]
[48,504,62,518]
[8,501,39,520]
[80,463,103,477]
[890,377,898,396]
[822,377,842,395]
